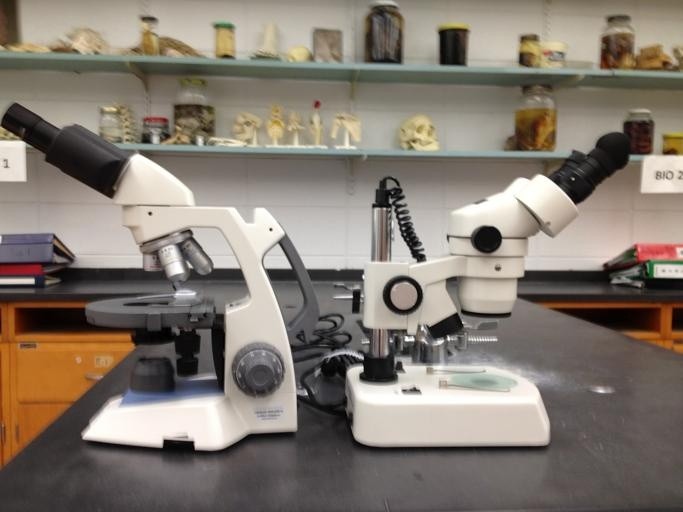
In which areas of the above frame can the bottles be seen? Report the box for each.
[142,117,170,144]
[137,16,159,57]
[173,77,216,145]
[519,33,541,68]
[662,131,683,155]
[623,109,654,154]
[213,21,237,59]
[514,83,557,151]
[600,15,635,70]
[366,0,404,64]
[439,24,469,64]
[98,106,123,143]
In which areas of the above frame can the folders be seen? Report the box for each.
[602,242,683,281]
[0,232,76,288]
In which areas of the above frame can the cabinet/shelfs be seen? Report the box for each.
[1,301,137,465]
[0,52,682,162]
[531,302,683,357]
